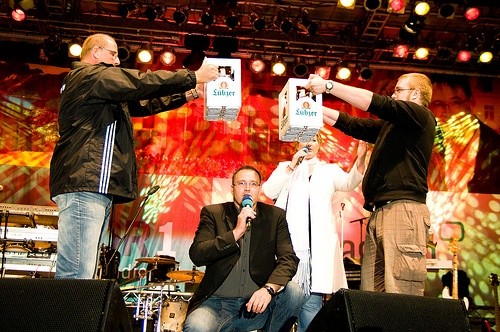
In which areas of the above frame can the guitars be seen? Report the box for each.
[449,237,459,300]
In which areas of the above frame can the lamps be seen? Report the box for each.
[7,0,500,80]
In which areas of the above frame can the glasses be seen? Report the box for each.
[393,87,413,92]
[233,181,260,188]
[97,47,118,59]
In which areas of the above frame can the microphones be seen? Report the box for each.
[241,195,253,229]
[142,186,160,200]
[296,146,309,165]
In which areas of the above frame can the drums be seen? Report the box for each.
[151,300,187,332]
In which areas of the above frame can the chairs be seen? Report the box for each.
[426,233,461,299]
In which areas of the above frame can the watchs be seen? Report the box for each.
[263,285,275,297]
[326,80,333,94]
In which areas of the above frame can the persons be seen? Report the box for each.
[49,33,220,282]
[262,133,367,332]
[425,73,500,194]
[183,166,305,332]
[304,72,437,296]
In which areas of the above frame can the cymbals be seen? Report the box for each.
[165,269,204,283]
[134,256,176,265]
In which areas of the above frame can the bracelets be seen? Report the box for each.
[190,89,199,99]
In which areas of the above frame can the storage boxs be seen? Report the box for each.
[279,78,324,143]
[207,57,242,121]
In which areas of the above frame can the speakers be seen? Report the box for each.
[0,277,133,332]
[305,288,472,332]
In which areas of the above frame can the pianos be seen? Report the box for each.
[0,203,59,273]
